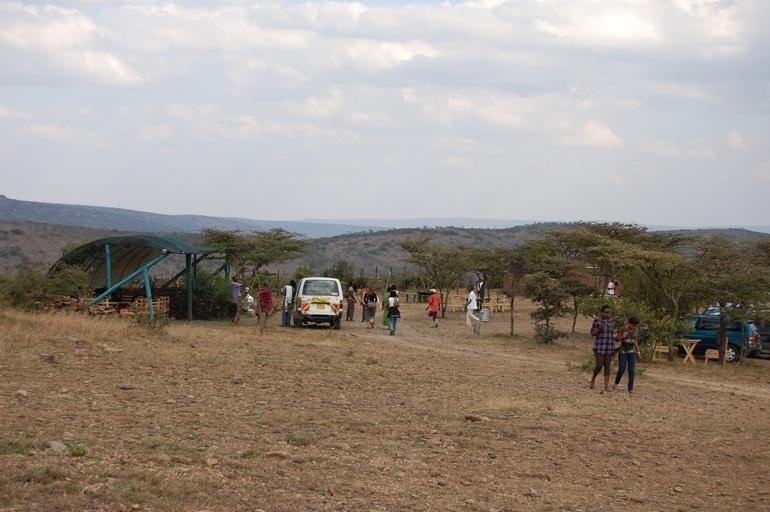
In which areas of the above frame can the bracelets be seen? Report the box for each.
[636,349,640,353]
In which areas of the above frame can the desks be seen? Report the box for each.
[679,338,702,365]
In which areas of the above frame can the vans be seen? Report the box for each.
[674,303,770,363]
[292,277,343,329]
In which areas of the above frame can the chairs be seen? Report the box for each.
[704,337,729,368]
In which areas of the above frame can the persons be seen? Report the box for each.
[607,277,616,295]
[346,282,401,336]
[464,285,480,331]
[279,278,297,327]
[231,275,245,324]
[589,304,614,391]
[255,282,273,323]
[426,288,441,328]
[613,280,620,295]
[611,316,643,393]
